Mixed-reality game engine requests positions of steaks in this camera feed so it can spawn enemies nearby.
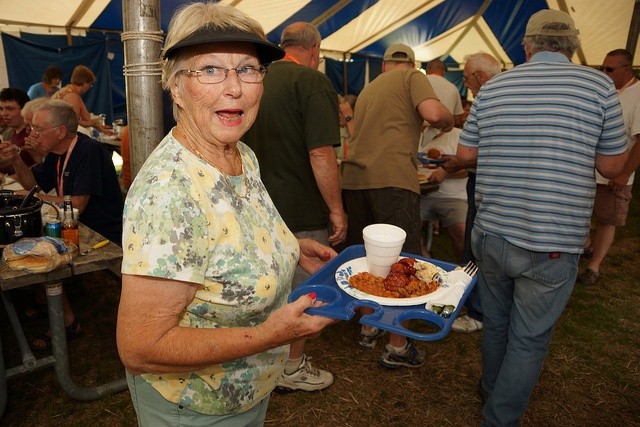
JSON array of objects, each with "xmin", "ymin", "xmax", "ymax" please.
[{"xmin": 349, "ymin": 272, "xmax": 407, "ymax": 298}]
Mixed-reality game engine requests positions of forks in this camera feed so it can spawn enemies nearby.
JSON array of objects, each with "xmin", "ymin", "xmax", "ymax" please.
[{"xmin": 442, "ymin": 261, "xmax": 478, "ymax": 317}]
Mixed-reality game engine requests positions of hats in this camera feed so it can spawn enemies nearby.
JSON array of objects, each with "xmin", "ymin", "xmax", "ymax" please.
[
  {"xmin": 525, "ymin": 10, "xmax": 580, "ymax": 37},
  {"xmin": 163, "ymin": 21, "xmax": 286, "ymax": 64},
  {"xmin": 383, "ymin": 42, "xmax": 414, "ymax": 63}
]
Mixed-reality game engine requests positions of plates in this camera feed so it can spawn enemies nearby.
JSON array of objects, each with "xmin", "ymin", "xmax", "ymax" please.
[{"xmin": 335, "ymin": 256, "xmax": 450, "ymax": 307}]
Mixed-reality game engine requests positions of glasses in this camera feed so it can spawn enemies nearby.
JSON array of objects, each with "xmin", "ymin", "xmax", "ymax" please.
[
  {"xmin": 31, "ymin": 125, "xmax": 60, "ymax": 135},
  {"xmin": 88, "ymin": 82, "xmax": 94, "ymax": 88},
  {"xmin": 175, "ymin": 64, "xmax": 269, "ymax": 83},
  {"xmin": 462, "ymin": 71, "xmax": 477, "ymax": 82},
  {"xmin": 600, "ymin": 64, "xmax": 630, "ymax": 72},
  {"xmin": 318, "ymin": 47, "xmax": 325, "ymax": 64}
]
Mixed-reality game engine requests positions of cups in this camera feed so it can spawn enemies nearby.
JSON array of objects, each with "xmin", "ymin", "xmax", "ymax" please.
[{"xmin": 362, "ymin": 223, "xmax": 406, "ymax": 279}]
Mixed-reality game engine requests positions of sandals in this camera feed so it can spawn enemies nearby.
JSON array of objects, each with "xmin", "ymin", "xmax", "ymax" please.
[
  {"xmin": 25, "ymin": 298, "xmax": 47, "ymax": 319},
  {"xmin": 583, "ymin": 269, "xmax": 598, "ymax": 285},
  {"xmin": 31, "ymin": 317, "xmax": 81, "ymax": 350}
]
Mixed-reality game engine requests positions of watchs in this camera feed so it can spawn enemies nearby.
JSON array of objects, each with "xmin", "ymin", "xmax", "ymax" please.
[{"xmin": 344, "ymin": 115, "xmax": 353, "ymax": 123}]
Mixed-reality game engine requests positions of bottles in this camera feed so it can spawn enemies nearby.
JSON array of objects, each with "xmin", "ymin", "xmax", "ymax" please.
[{"xmin": 61, "ymin": 195, "xmax": 79, "ymax": 251}]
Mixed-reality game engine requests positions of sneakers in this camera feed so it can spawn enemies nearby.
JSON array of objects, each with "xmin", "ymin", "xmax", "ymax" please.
[
  {"xmin": 273, "ymin": 354, "xmax": 333, "ymax": 393},
  {"xmin": 380, "ymin": 341, "xmax": 427, "ymax": 369},
  {"xmin": 359, "ymin": 326, "xmax": 386, "ymax": 347},
  {"xmin": 451, "ymin": 316, "xmax": 483, "ymax": 333}
]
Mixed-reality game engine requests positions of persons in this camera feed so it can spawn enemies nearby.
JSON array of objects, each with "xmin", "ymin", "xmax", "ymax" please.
[
  {"xmin": 444, "ymin": 54, "xmax": 501, "ymax": 333},
  {"xmin": 1, "ymin": 99, "xmax": 124, "ymax": 350},
  {"xmin": 50, "ymin": 65, "xmax": 112, "ymax": 137},
  {"xmin": 423, "ymin": 59, "xmax": 464, "ymax": 127},
  {"xmin": 339, "ymin": 45, "xmax": 455, "ymax": 366},
  {"xmin": 336, "ymin": 93, "xmax": 357, "ymax": 138},
  {"xmin": 461, "ymin": 9, "xmax": 629, "ymax": 426},
  {"xmin": 461, "ymin": 100, "xmax": 473, "ymax": 121},
  {"xmin": 577, "ymin": 50, "xmax": 638, "ymax": 293},
  {"xmin": 27, "ymin": 65, "xmax": 64, "ymax": 100},
  {"xmin": 1, "ymin": 87, "xmax": 33, "ymax": 165},
  {"xmin": 238, "ymin": 21, "xmax": 348, "ymax": 391},
  {"xmin": 115, "ymin": 3, "xmax": 344, "ymax": 427},
  {"xmin": 23, "ymin": 98, "xmax": 50, "ymax": 161},
  {"xmin": 417, "ymin": 126, "xmax": 469, "ymax": 265}
]
